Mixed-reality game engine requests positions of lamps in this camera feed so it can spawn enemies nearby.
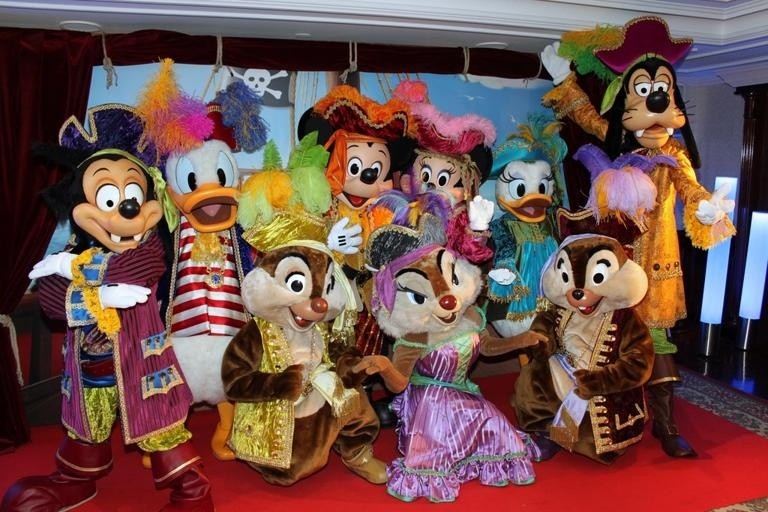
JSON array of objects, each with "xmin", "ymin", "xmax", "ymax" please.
[{"xmin": 699, "ymin": 173, "xmax": 768, "ymax": 358}]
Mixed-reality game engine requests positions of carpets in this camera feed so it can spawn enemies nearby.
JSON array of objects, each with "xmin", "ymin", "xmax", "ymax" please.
[{"xmin": 0, "ymin": 359, "xmax": 767, "ymax": 510}]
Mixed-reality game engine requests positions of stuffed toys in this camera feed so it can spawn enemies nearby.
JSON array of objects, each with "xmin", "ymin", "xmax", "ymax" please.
[{"xmin": 0, "ymin": 14, "xmax": 737, "ymax": 512}]
